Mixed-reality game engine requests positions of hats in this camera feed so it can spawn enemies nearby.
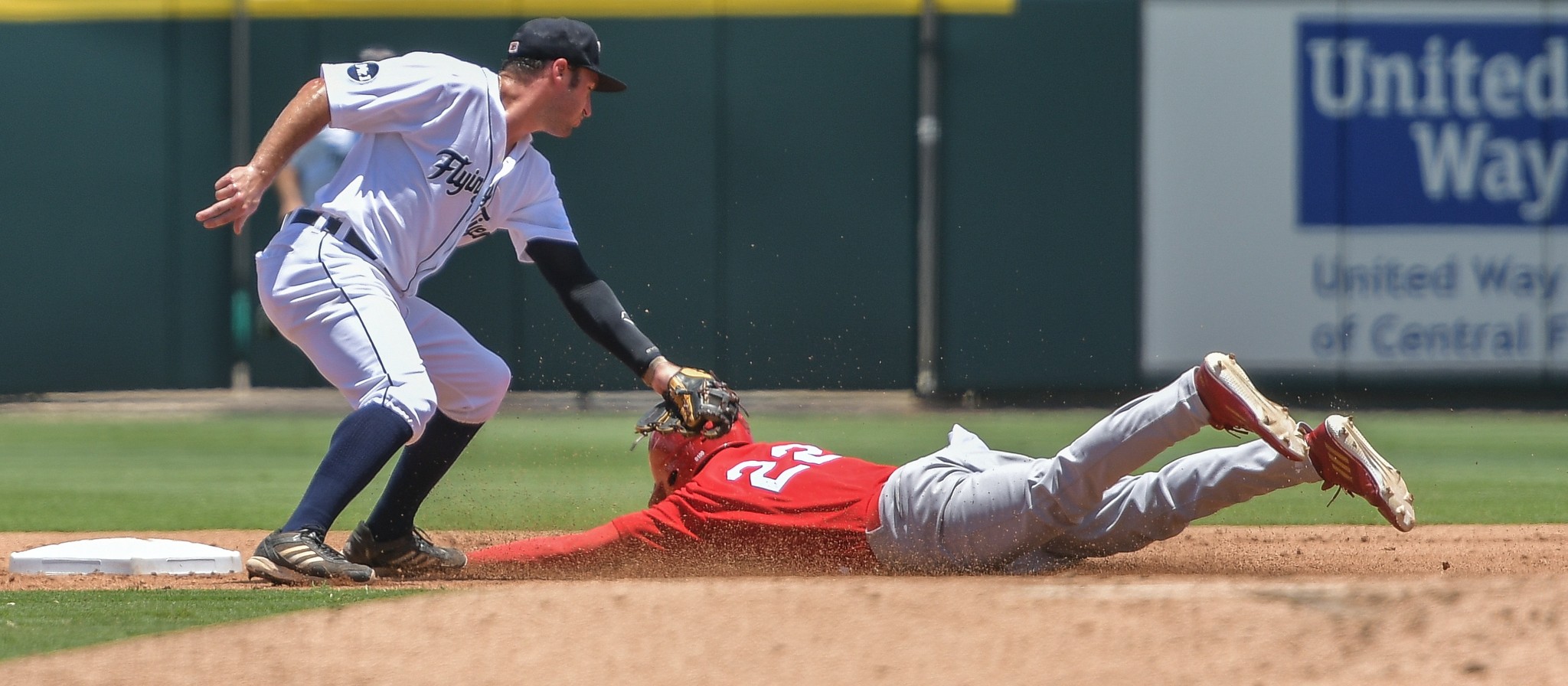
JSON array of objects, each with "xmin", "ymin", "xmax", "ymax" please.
[{"xmin": 509, "ymin": 14, "xmax": 626, "ymax": 93}]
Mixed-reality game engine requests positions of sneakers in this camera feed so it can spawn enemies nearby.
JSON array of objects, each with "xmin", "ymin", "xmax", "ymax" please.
[
  {"xmin": 1303, "ymin": 413, "xmax": 1415, "ymax": 530},
  {"xmin": 342, "ymin": 517, "xmax": 468, "ymax": 571},
  {"xmin": 1195, "ymin": 352, "xmax": 1310, "ymax": 462},
  {"xmin": 245, "ymin": 531, "xmax": 377, "ymax": 585}
]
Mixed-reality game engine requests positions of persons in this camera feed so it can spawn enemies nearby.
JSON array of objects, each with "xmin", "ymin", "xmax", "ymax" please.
[
  {"xmin": 194, "ymin": 15, "xmax": 737, "ymax": 585},
  {"xmin": 441, "ymin": 352, "xmax": 1416, "ymax": 582}
]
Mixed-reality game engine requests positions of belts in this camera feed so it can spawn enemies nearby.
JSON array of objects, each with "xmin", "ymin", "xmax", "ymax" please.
[
  {"xmin": 292, "ymin": 209, "xmax": 376, "ymax": 262},
  {"xmin": 864, "ymin": 484, "xmax": 883, "ymax": 532}
]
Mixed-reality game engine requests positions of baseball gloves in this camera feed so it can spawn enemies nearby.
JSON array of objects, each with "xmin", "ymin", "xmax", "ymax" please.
[{"xmin": 635, "ymin": 367, "xmax": 740, "ymax": 440}]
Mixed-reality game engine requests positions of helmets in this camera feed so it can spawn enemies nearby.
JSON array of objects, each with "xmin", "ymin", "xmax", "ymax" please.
[{"xmin": 648, "ymin": 403, "xmax": 751, "ymax": 507}]
